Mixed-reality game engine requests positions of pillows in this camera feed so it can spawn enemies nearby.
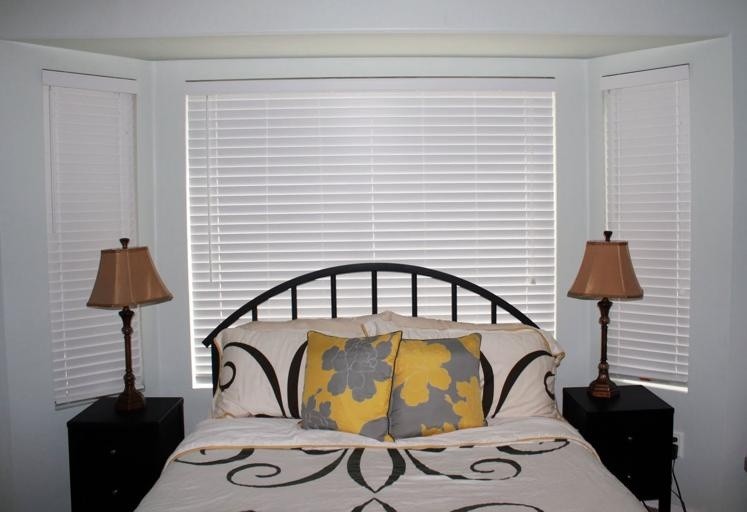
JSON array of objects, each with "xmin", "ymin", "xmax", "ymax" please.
[
  {"xmin": 387, "ymin": 331, "xmax": 488, "ymax": 440},
  {"xmin": 209, "ymin": 310, "xmax": 391, "ymax": 419},
  {"xmin": 390, "ymin": 312, "xmax": 566, "ymax": 420},
  {"xmin": 299, "ymin": 330, "xmax": 404, "ymax": 443}
]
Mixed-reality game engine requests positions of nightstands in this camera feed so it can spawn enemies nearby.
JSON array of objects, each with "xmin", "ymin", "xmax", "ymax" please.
[
  {"xmin": 563, "ymin": 384, "xmax": 675, "ymax": 512},
  {"xmin": 66, "ymin": 397, "xmax": 186, "ymax": 512}
]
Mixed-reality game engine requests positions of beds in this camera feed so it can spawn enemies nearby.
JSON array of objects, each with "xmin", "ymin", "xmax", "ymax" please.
[{"xmin": 134, "ymin": 262, "xmax": 653, "ymax": 512}]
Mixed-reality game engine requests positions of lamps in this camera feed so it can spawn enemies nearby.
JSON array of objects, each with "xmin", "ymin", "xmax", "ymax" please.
[
  {"xmin": 85, "ymin": 238, "xmax": 174, "ymax": 414},
  {"xmin": 567, "ymin": 231, "xmax": 645, "ymax": 399}
]
